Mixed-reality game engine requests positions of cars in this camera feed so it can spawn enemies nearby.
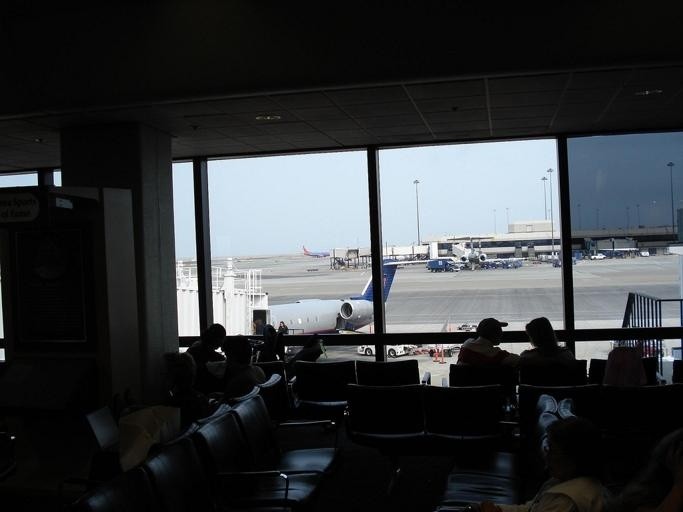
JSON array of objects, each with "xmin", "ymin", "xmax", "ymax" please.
[
  {"xmin": 357, "ymin": 340, "xmax": 416, "ymax": 358},
  {"xmin": 428, "ymin": 344, "xmax": 456, "ymax": 357}
]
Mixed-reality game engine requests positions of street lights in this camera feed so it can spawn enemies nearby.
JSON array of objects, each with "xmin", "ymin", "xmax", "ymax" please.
[
  {"xmin": 627, "ymin": 207, "xmax": 630, "ymax": 228},
  {"xmin": 542, "ymin": 176, "xmax": 549, "ymax": 221},
  {"xmin": 597, "ymin": 208, "xmax": 600, "ymax": 230},
  {"xmin": 667, "ymin": 160, "xmax": 676, "ymax": 234},
  {"xmin": 637, "ymin": 204, "xmax": 641, "ymax": 226},
  {"xmin": 578, "ymin": 203, "xmax": 581, "ymax": 231},
  {"xmin": 547, "ymin": 167, "xmax": 554, "ymax": 258},
  {"xmin": 413, "ymin": 179, "xmax": 422, "ymax": 248}
]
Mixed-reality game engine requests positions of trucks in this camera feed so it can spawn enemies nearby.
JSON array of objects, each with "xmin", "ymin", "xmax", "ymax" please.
[{"xmin": 429, "ymin": 259, "xmax": 460, "ymax": 272}]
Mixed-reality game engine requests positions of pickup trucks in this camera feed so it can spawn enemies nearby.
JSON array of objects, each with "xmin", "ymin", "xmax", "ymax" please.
[{"xmin": 592, "ymin": 254, "xmax": 606, "ymax": 260}]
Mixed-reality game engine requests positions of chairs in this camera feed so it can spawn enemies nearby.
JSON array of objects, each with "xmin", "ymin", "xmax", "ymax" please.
[
  {"xmin": 232, "ymin": 385, "xmax": 260, "ymax": 403},
  {"xmin": 196, "ymin": 403, "xmax": 235, "ymax": 422},
  {"xmin": 448, "ymin": 361, "xmax": 516, "ymax": 384},
  {"xmin": 672, "ymin": 355, "xmax": 683, "ymax": 385},
  {"xmin": 198, "ymin": 411, "xmax": 320, "ymax": 505},
  {"xmin": 161, "ymin": 421, "xmax": 200, "ymax": 448},
  {"xmin": 257, "ymin": 371, "xmax": 284, "ymax": 390},
  {"xmin": 346, "ymin": 381, "xmax": 433, "ymax": 434},
  {"xmin": 516, "ymin": 357, "xmax": 586, "ymax": 383},
  {"xmin": 588, "ymin": 355, "xmax": 661, "ymax": 383},
  {"xmin": 235, "ymin": 394, "xmax": 339, "ymax": 473},
  {"xmin": 294, "ymin": 359, "xmax": 356, "ymax": 409},
  {"xmin": 431, "ymin": 382, "xmax": 508, "ymax": 433},
  {"xmin": 518, "ymin": 381, "xmax": 600, "ymax": 438},
  {"xmin": 85, "ymin": 458, "xmax": 175, "ymax": 512},
  {"xmin": 146, "ymin": 430, "xmax": 293, "ymax": 512},
  {"xmin": 601, "ymin": 382, "xmax": 683, "ymax": 439},
  {"xmin": 356, "ymin": 359, "xmax": 432, "ymax": 388},
  {"xmin": 344, "ymin": 429, "xmax": 429, "ymax": 493}
]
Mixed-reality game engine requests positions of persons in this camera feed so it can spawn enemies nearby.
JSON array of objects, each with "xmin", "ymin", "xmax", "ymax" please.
[
  {"xmin": 181, "ymin": 323, "xmax": 226, "ymax": 367},
  {"xmin": 465, "ymin": 417, "xmax": 612, "ymax": 512},
  {"xmin": 253, "ymin": 319, "xmax": 265, "ymax": 333},
  {"xmin": 457, "ymin": 318, "xmax": 519, "ymax": 379},
  {"xmin": 521, "ymin": 317, "xmax": 578, "ymax": 387},
  {"xmin": 278, "ymin": 321, "xmax": 289, "ymax": 335},
  {"xmin": 263, "ymin": 324, "xmax": 285, "ymax": 361},
  {"xmin": 222, "ymin": 335, "xmax": 266, "ymax": 397}
]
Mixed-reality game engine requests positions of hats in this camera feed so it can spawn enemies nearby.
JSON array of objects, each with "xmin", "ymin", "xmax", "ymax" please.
[{"xmin": 476, "ymin": 317, "xmax": 508, "ymax": 333}]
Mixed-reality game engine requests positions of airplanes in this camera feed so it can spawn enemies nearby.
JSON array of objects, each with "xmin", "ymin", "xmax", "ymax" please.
[
  {"xmin": 384, "ymin": 248, "xmax": 528, "ymax": 273},
  {"xmin": 303, "ymin": 248, "xmax": 329, "ymax": 260},
  {"xmin": 252, "ymin": 258, "xmax": 398, "ymax": 354}
]
